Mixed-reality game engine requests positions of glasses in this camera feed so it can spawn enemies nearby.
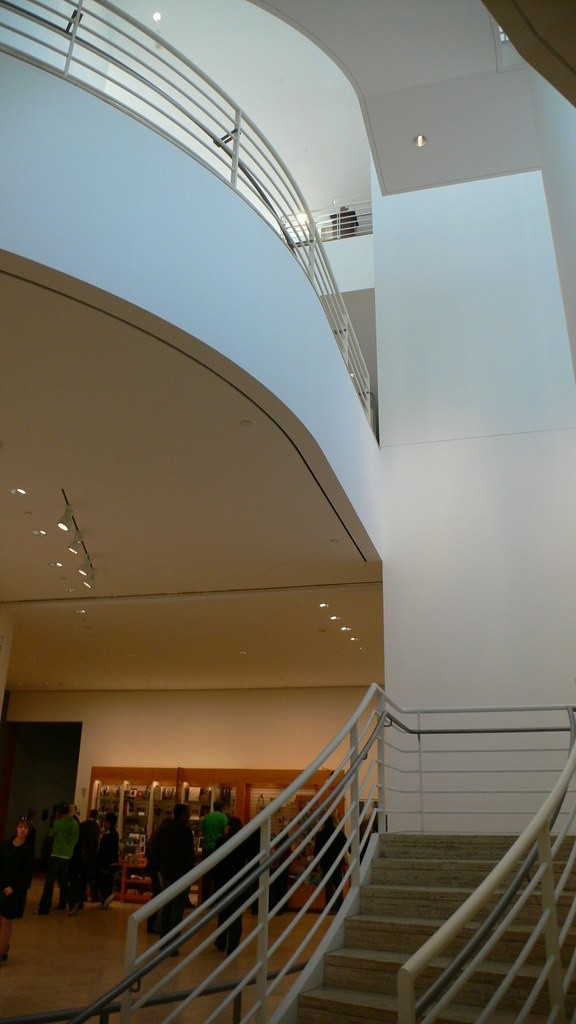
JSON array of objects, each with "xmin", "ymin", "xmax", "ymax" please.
[{"xmin": 18, "ymin": 816, "xmax": 33, "ymax": 825}]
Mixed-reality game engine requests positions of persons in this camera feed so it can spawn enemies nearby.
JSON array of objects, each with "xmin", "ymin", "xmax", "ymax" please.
[
  {"xmin": 38, "ymin": 801, "xmax": 81, "ymax": 913},
  {"xmin": 349, "ymin": 820, "xmax": 380, "ymax": 864},
  {"xmin": 157, "ymin": 804, "xmax": 194, "ymax": 956},
  {"xmin": 214, "ymin": 817, "xmax": 252, "ymax": 957},
  {"xmin": 199, "ymin": 800, "xmax": 229, "ymax": 910},
  {"xmin": 315, "ymin": 816, "xmax": 352, "ymax": 913},
  {"xmin": 79, "ymin": 809, "xmax": 102, "ymax": 903},
  {"xmin": 0, "ymin": 816, "xmax": 36, "ymax": 961},
  {"xmin": 89, "ymin": 813, "xmax": 119, "ymax": 907}
]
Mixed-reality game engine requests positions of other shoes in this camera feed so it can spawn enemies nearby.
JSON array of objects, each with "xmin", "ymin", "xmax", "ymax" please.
[
  {"xmin": 78, "ymin": 907, "xmax": 83, "ymax": 912},
  {"xmin": 214, "ymin": 939, "xmax": 230, "ymax": 958},
  {"xmin": 185, "ymin": 902, "xmax": 196, "ymax": 907},
  {"xmin": 327, "ymin": 907, "xmax": 339, "ymax": 915},
  {"xmin": 164, "ymin": 949, "xmax": 179, "ymax": 956},
  {"xmin": 69, "ymin": 901, "xmax": 79, "ymax": 915},
  {"xmin": 51, "ymin": 906, "xmax": 65, "ymax": 911},
  {"xmin": 251, "ymin": 906, "xmax": 282, "ymax": 916},
  {"xmin": 100, "ymin": 893, "xmax": 115, "ymax": 908},
  {"xmin": 34, "ymin": 910, "xmax": 39, "ymax": 914},
  {"xmin": 146, "ymin": 926, "xmax": 161, "ymax": 933}
]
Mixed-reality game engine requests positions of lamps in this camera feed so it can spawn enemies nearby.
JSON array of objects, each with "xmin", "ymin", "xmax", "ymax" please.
[{"xmin": 56, "ymin": 487, "xmax": 96, "ymax": 589}]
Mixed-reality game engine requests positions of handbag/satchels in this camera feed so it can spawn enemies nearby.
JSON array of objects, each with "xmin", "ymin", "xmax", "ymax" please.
[{"xmin": 343, "ymin": 848, "xmax": 351, "ymax": 865}]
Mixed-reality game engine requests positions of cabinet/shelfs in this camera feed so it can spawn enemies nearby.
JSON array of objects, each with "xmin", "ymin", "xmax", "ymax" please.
[{"xmin": 84, "ymin": 778, "xmax": 222, "ymax": 911}]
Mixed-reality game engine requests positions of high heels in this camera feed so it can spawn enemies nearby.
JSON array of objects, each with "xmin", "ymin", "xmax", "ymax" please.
[{"xmin": 0, "ymin": 944, "xmax": 9, "ymax": 960}]
